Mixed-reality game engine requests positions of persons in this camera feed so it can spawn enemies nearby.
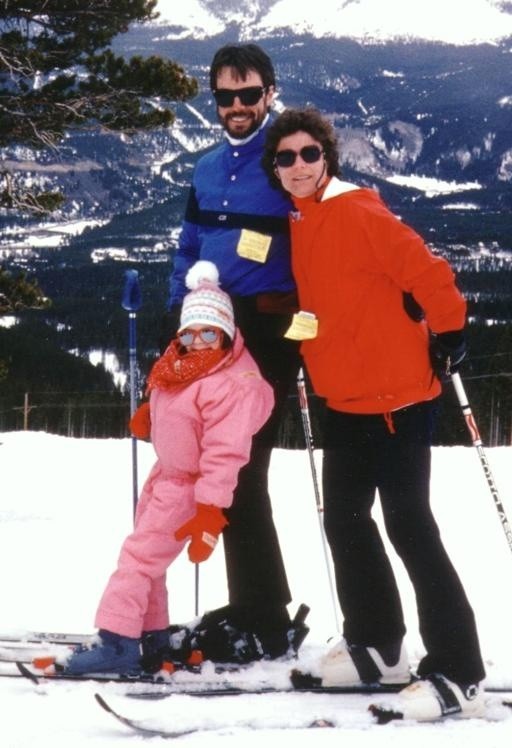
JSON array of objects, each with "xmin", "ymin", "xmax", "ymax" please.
[
  {"xmin": 260, "ymin": 108, "xmax": 484, "ymax": 717},
  {"xmin": 66, "ymin": 261, "xmax": 276, "ymax": 673},
  {"xmin": 169, "ymin": 43, "xmax": 296, "ymax": 652}
]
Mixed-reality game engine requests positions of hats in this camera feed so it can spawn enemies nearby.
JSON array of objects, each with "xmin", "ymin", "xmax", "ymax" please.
[{"xmin": 178, "ymin": 259, "xmax": 237, "ymax": 343}]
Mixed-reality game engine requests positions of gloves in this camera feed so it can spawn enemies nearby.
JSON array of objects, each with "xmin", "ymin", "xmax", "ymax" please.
[
  {"xmin": 174, "ymin": 501, "xmax": 231, "ymax": 567},
  {"xmin": 127, "ymin": 402, "xmax": 153, "ymax": 444},
  {"xmin": 425, "ymin": 327, "xmax": 472, "ymax": 382}
]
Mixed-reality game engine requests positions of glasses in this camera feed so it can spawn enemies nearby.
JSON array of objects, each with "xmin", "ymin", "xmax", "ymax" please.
[
  {"xmin": 270, "ymin": 143, "xmax": 332, "ymax": 167},
  {"xmin": 211, "ymin": 86, "xmax": 268, "ymax": 108},
  {"xmin": 177, "ymin": 328, "xmax": 226, "ymax": 347}
]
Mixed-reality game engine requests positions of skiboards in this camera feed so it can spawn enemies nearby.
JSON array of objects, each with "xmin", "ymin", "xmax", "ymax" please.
[
  {"xmin": 0, "ymin": 634, "xmax": 512, "ymax": 736},
  {"xmin": 94, "ymin": 673, "xmax": 511, "ymax": 737},
  {"xmin": 1, "ymin": 634, "xmax": 504, "ymax": 682}
]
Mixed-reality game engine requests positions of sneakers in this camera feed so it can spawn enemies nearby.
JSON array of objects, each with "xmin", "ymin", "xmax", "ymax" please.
[
  {"xmin": 290, "ymin": 636, "xmax": 414, "ymax": 692},
  {"xmin": 368, "ymin": 671, "xmax": 488, "ymax": 723},
  {"xmin": 138, "ymin": 624, "xmax": 176, "ymax": 669},
  {"xmin": 60, "ymin": 629, "xmax": 143, "ymax": 676}
]
